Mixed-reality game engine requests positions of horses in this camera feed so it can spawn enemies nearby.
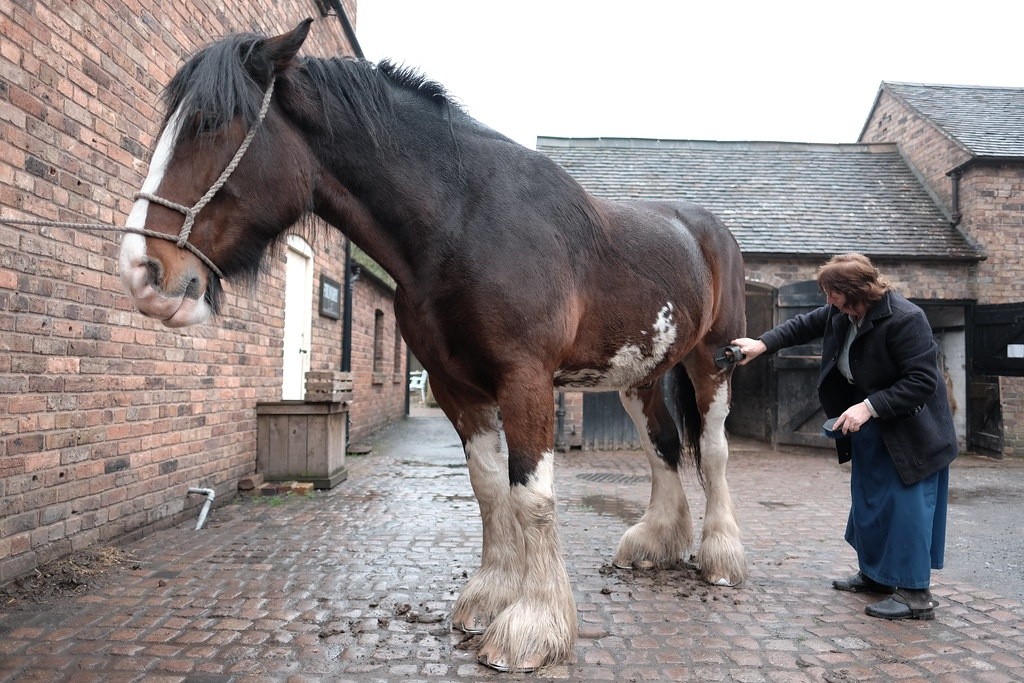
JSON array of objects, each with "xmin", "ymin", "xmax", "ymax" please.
[{"xmin": 115, "ymin": 16, "xmax": 748, "ymax": 672}]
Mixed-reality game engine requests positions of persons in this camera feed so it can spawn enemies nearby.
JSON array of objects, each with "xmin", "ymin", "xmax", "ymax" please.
[{"xmin": 723, "ymin": 254, "xmax": 960, "ymax": 621}]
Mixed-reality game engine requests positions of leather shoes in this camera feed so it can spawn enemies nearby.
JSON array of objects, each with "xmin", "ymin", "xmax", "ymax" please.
[
  {"xmin": 864, "ymin": 587, "xmax": 936, "ymax": 621},
  {"xmin": 832, "ymin": 570, "xmax": 896, "ymax": 596}
]
